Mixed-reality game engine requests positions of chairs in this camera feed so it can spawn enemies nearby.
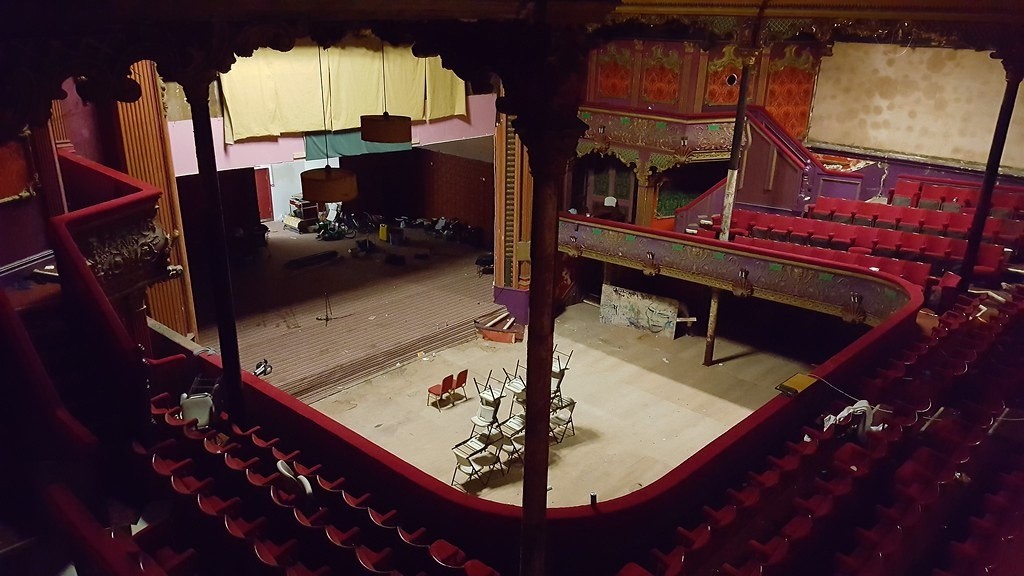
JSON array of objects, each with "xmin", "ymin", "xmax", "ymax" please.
[{"xmin": 107, "ymin": 180, "xmax": 1024, "ymax": 576}]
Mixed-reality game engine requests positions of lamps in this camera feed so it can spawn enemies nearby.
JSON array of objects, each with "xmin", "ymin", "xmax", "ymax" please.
[
  {"xmin": 361, "ymin": 41, "xmax": 412, "ymax": 144},
  {"xmin": 301, "ymin": 46, "xmax": 358, "ymax": 203}
]
